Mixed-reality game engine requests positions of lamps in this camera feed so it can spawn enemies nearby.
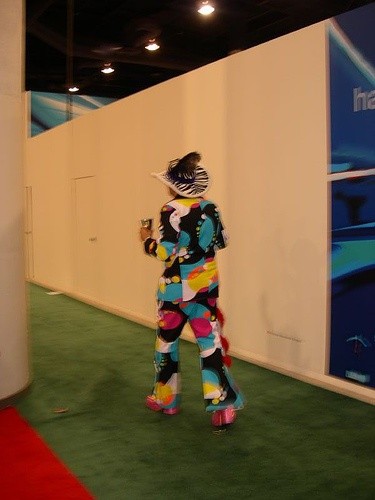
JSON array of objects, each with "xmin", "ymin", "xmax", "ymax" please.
[
  {"xmin": 197, "ymin": 0, "xmax": 216, "ymax": 17},
  {"xmin": 145, "ymin": 38, "xmax": 161, "ymax": 51},
  {"xmin": 68, "ymin": 81, "xmax": 80, "ymax": 93},
  {"xmin": 100, "ymin": 60, "xmax": 115, "ymax": 75}
]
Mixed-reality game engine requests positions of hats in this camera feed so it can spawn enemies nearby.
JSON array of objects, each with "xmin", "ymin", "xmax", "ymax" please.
[{"xmin": 150, "ymin": 150, "xmax": 211, "ymax": 198}]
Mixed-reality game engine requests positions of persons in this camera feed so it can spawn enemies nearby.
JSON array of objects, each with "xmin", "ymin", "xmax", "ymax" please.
[{"xmin": 141, "ymin": 146, "xmax": 246, "ymax": 430}]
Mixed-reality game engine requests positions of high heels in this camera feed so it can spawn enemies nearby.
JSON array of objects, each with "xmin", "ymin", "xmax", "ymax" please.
[
  {"xmin": 212, "ymin": 405, "xmax": 237, "ymax": 427},
  {"xmin": 145, "ymin": 394, "xmax": 180, "ymax": 414}
]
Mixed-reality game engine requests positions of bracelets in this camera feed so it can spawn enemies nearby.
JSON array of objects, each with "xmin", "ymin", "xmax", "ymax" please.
[{"xmin": 142, "ymin": 236, "xmax": 151, "ymax": 244}]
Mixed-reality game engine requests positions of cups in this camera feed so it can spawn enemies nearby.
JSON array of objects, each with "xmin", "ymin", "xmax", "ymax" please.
[{"xmin": 141, "ymin": 218, "xmax": 153, "ymax": 232}]
